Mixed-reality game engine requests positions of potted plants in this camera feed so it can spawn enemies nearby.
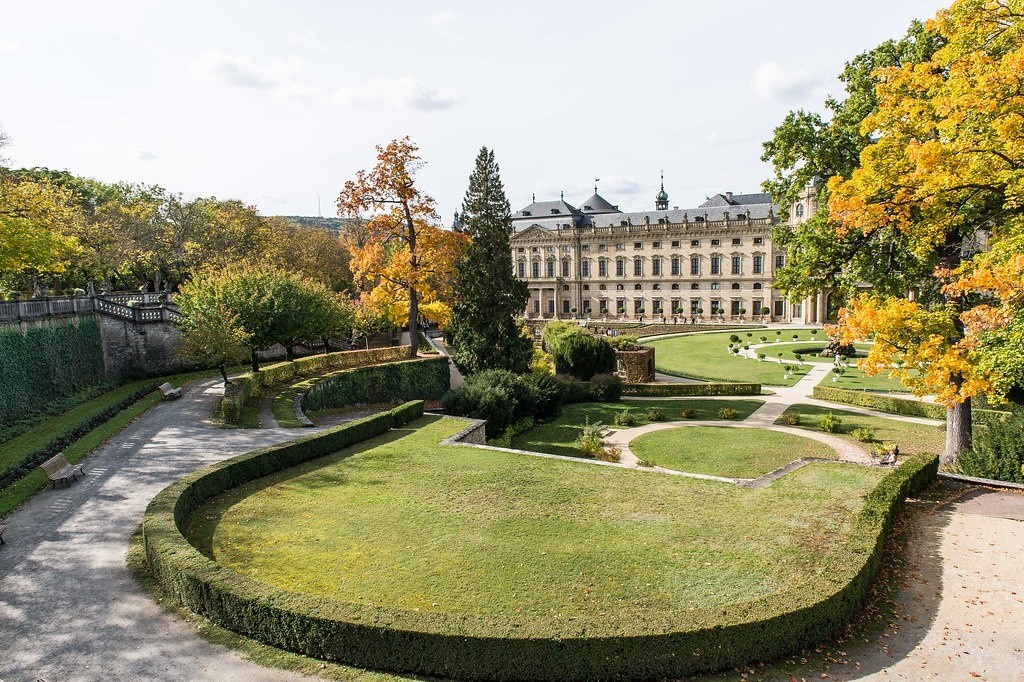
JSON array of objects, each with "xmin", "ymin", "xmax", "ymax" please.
[
  {"xmin": 658, "ymin": 309, "xmax": 664, "ymax": 322},
  {"xmin": 792, "ymin": 335, "xmax": 798, "ymax": 341},
  {"xmin": 601, "ymin": 308, "xmax": 607, "ymax": 322},
  {"xmin": 784, "ymin": 353, "xmax": 805, "ymax": 380},
  {"xmin": 757, "ymin": 353, "xmax": 766, "ymax": 362},
  {"xmin": 718, "ymin": 309, "xmax": 725, "ymax": 322},
  {"xmin": 776, "ymin": 353, "xmax": 783, "ymax": 365},
  {"xmin": 619, "ymin": 307, "xmax": 625, "ymax": 323},
  {"xmin": 729, "ymin": 332, "xmax": 752, "ymax": 359},
  {"xmin": 760, "ymin": 307, "xmax": 770, "ymax": 324},
  {"xmin": 571, "ymin": 307, "xmax": 578, "ymax": 320},
  {"xmin": 638, "ymin": 308, "xmax": 645, "ymax": 321},
  {"xmin": 585, "ymin": 307, "xmax": 592, "ymax": 321},
  {"xmin": 810, "ymin": 329, "xmax": 817, "ymax": 340},
  {"xmin": 775, "ymin": 331, "xmax": 781, "ymax": 342},
  {"xmin": 832, "ymin": 355, "xmax": 849, "ymax": 383},
  {"xmin": 675, "ymin": 307, "xmax": 683, "ymax": 323},
  {"xmin": 760, "ymin": 336, "xmax": 766, "ymax": 344},
  {"xmin": 696, "ymin": 308, "xmax": 703, "ymax": 323},
  {"xmin": 738, "ymin": 308, "xmax": 746, "ymax": 323}
]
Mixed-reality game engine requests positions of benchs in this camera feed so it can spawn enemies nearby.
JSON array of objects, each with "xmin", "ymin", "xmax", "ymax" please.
[
  {"xmin": 38, "ymin": 451, "xmax": 86, "ymax": 490},
  {"xmin": 157, "ymin": 382, "xmax": 183, "ymax": 404}
]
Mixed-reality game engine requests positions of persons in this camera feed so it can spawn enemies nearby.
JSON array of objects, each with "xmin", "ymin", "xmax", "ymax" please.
[
  {"xmin": 577, "ymin": 321, "xmax": 623, "ymax": 336},
  {"xmin": 422, "ymin": 320, "xmax": 429, "ymax": 337},
  {"xmin": 633, "ymin": 314, "xmax": 695, "ymax": 324},
  {"xmin": 880, "ymin": 445, "xmax": 899, "ymax": 465},
  {"xmin": 833, "ymin": 348, "xmax": 840, "ymax": 367}
]
